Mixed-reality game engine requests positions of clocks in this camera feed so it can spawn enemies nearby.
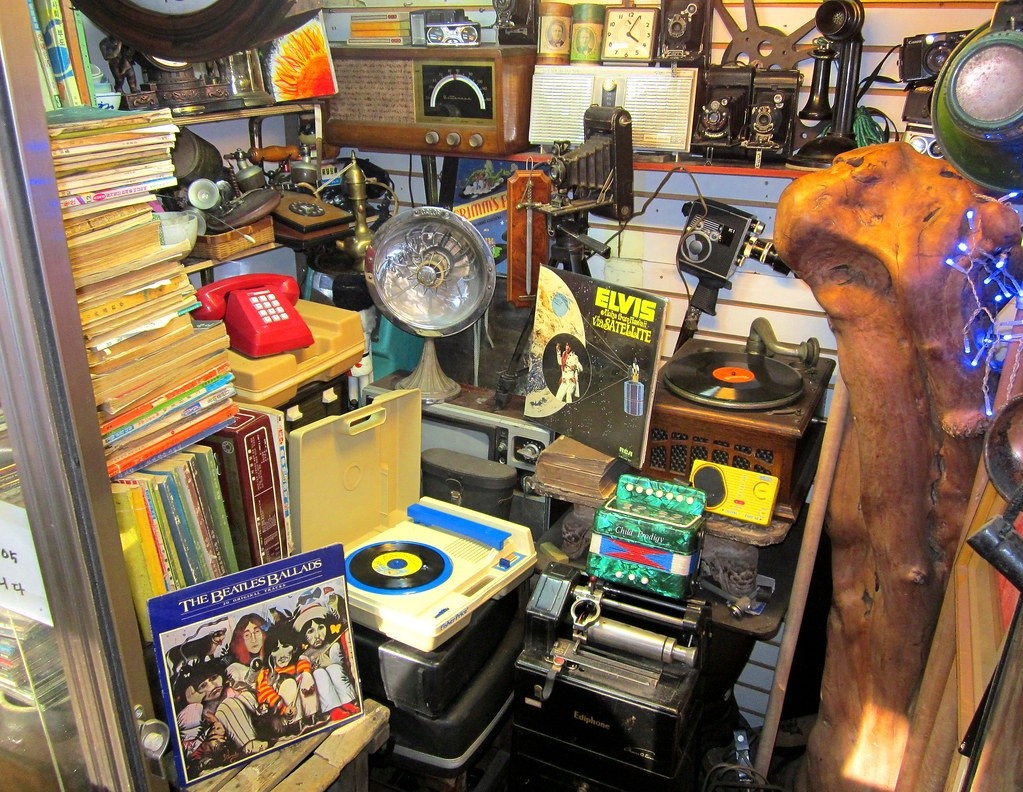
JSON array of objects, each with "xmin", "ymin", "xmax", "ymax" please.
[{"xmin": 600, "ymin": 6, "xmax": 661, "ymax": 63}]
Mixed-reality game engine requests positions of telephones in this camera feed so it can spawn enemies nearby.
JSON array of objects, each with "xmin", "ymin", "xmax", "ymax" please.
[{"xmin": 193, "ymin": 272, "xmax": 315, "ymax": 359}]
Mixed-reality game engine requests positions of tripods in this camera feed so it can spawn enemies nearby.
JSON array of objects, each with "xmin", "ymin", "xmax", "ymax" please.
[{"xmin": 491, "ymin": 199, "xmax": 617, "ymax": 407}]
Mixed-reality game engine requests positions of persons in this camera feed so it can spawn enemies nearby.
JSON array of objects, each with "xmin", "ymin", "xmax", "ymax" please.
[
  {"xmin": 555, "ymin": 338, "xmax": 583, "ymax": 404},
  {"xmin": 548, "ymin": 24, "xmax": 564, "ymax": 48},
  {"xmin": 577, "ymin": 29, "xmax": 592, "ymax": 55}
]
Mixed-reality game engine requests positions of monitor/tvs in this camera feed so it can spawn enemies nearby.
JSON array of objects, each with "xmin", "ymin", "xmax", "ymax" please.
[{"xmin": 358, "ymin": 368, "xmax": 559, "ymax": 545}]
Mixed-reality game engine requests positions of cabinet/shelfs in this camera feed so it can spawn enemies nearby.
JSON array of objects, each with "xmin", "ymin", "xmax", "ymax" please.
[{"xmin": 170, "ymin": 98, "xmax": 324, "ymax": 275}]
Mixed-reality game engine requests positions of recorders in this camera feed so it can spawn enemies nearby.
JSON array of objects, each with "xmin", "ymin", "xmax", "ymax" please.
[
  {"xmin": 317, "ymin": 46, "xmax": 537, "ymax": 158},
  {"xmin": 528, "ymin": 65, "xmax": 705, "ymax": 162},
  {"xmin": 423, "ymin": 23, "xmax": 482, "ymax": 47}
]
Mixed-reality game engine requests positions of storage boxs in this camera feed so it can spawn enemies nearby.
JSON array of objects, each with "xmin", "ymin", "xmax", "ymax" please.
[
  {"xmin": 420, "ymin": 448, "xmax": 518, "ymax": 521},
  {"xmin": 228, "ymin": 298, "xmax": 367, "ymax": 408}
]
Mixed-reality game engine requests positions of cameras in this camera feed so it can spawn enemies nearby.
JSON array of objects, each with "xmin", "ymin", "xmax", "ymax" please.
[
  {"xmin": 753, "ymin": 67, "xmax": 804, "ymax": 168},
  {"xmin": 902, "ymin": 124, "xmax": 944, "ymax": 160},
  {"xmin": 676, "ymin": 197, "xmax": 790, "ymax": 316},
  {"xmin": 661, "ymin": 0, "xmax": 714, "ymax": 68},
  {"xmin": 900, "ymin": 27, "xmax": 977, "ymax": 87},
  {"xmin": 691, "ymin": 62, "xmax": 753, "ymax": 168},
  {"xmin": 492, "ymin": 0, "xmax": 540, "ymax": 45},
  {"xmin": 531, "ymin": 103, "xmax": 634, "ymax": 223},
  {"xmin": 902, "ymin": 86, "xmax": 939, "ymax": 123}
]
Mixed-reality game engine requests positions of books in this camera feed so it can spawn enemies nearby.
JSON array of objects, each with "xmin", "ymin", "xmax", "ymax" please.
[
  {"xmin": 27, "ymin": 0, "xmax": 98, "ymax": 112},
  {"xmin": 533, "ymin": 434, "xmax": 627, "ymax": 498},
  {"xmin": 265, "ymin": 15, "xmax": 339, "ymax": 104},
  {"xmin": 62, "ymin": 194, "xmax": 241, "ymax": 482},
  {"xmin": 46, "ymin": 105, "xmax": 179, "ymax": 209},
  {"xmin": 0, "ymin": 412, "xmax": 25, "ymax": 508},
  {"xmin": 0, "ymin": 607, "xmax": 68, "ymax": 712},
  {"xmin": 522, "ymin": 263, "xmax": 669, "ymax": 471},
  {"xmin": 110, "ymin": 402, "xmax": 293, "ymax": 645},
  {"xmin": 346, "ymin": 12, "xmax": 411, "ymax": 46}
]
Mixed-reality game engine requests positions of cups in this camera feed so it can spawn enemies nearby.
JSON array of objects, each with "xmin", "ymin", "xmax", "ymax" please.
[{"xmin": 94, "ymin": 83, "xmax": 111, "ymax": 92}]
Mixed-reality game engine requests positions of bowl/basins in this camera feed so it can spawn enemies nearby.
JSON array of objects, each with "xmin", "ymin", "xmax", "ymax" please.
[
  {"xmin": 89, "ymin": 63, "xmax": 104, "ymax": 85},
  {"xmin": 95, "ymin": 92, "xmax": 121, "ymax": 110},
  {"xmin": 153, "ymin": 211, "xmax": 198, "ymax": 263}
]
